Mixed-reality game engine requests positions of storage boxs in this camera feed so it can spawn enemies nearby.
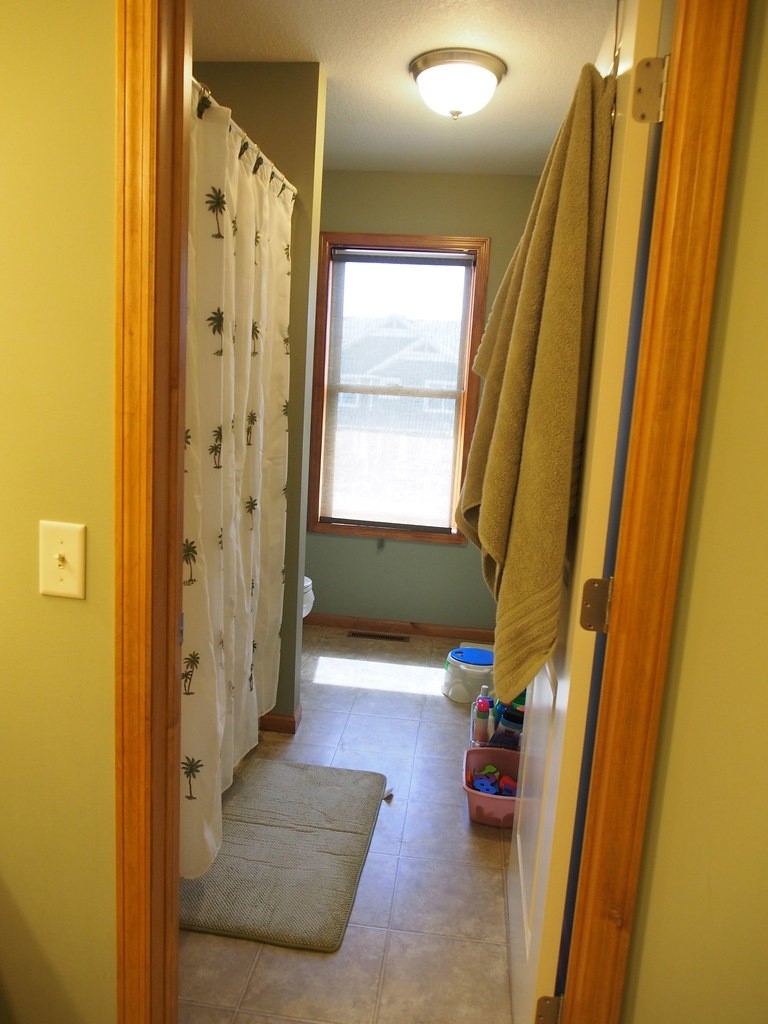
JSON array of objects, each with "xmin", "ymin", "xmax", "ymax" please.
[
  {"xmin": 462, "ymin": 746, "xmax": 525, "ymax": 828},
  {"xmin": 470, "ymin": 702, "xmax": 522, "ymax": 751}
]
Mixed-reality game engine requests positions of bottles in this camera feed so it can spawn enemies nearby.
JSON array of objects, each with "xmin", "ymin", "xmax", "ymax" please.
[{"xmin": 473, "ymin": 684, "xmax": 524, "ymax": 745}]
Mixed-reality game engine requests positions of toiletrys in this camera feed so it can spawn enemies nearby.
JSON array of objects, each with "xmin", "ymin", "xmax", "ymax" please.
[{"xmin": 473, "ymin": 684, "xmax": 526, "ymax": 746}]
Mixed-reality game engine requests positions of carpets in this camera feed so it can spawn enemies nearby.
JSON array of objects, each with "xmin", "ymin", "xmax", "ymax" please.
[{"xmin": 170, "ymin": 756, "xmax": 388, "ymax": 953}]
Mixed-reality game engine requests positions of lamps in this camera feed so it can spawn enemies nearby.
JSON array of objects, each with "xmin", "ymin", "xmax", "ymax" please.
[{"xmin": 409, "ymin": 47, "xmax": 506, "ymax": 122}]
